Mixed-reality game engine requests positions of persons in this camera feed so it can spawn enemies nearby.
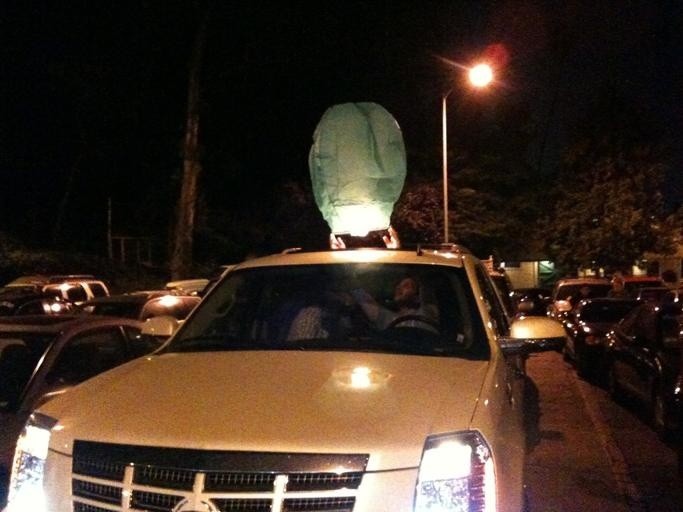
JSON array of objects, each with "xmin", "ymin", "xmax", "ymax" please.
[
  {"xmin": 571, "ymin": 283, "xmax": 595, "ymax": 306},
  {"xmin": 605, "ymin": 274, "xmax": 632, "ymax": 300},
  {"xmin": 330, "ymin": 225, "xmax": 439, "ymax": 334}
]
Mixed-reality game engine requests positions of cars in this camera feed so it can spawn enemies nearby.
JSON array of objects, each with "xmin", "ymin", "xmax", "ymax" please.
[
  {"xmin": 1, "ymin": 312, "xmax": 170, "ymax": 500},
  {"xmin": 1, "ymin": 269, "xmax": 223, "ymax": 349},
  {"xmin": 600, "ymin": 292, "xmax": 682, "ymax": 439},
  {"xmin": 482, "ymin": 255, "xmax": 682, "ymax": 325},
  {"xmin": 557, "ymin": 297, "xmax": 641, "ymax": 380}
]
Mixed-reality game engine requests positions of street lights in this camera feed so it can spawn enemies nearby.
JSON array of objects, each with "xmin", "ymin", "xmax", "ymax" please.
[{"xmin": 440, "ymin": 58, "xmax": 500, "ymax": 251}]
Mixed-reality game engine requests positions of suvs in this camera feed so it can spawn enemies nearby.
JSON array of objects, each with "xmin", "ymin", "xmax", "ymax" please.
[{"xmin": 1, "ymin": 240, "xmax": 569, "ymax": 512}]
